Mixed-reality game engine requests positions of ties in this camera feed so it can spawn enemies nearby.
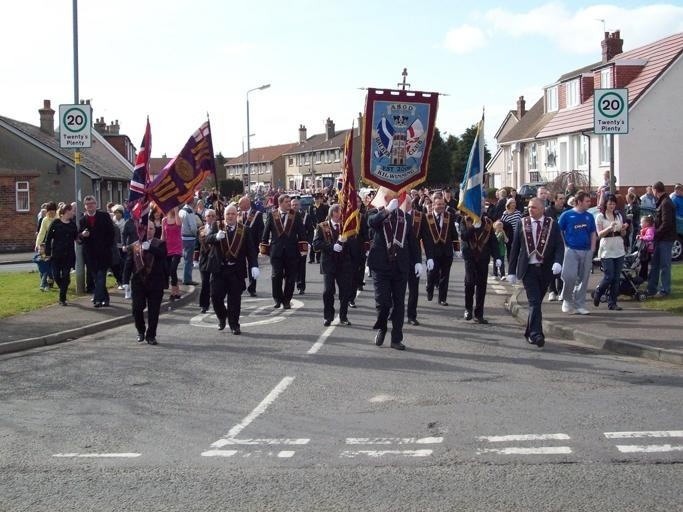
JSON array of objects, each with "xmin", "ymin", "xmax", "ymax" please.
[
  {"xmin": 278, "ymin": 212, "xmax": 287, "ymax": 226},
  {"xmin": 434, "ymin": 212, "xmax": 443, "ymax": 225},
  {"xmin": 333, "ymin": 223, "xmax": 341, "ymax": 234},
  {"xmin": 532, "ymin": 220, "xmax": 542, "ymax": 247}
]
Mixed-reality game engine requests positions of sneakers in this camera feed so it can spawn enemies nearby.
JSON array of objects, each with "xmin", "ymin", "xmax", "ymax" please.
[
  {"xmin": 573, "ymin": 307, "xmax": 592, "ymax": 316},
  {"xmin": 607, "ymin": 303, "xmax": 623, "ymax": 312},
  {"xmin": 547, "ymin": 292, "xmax": 556, "ymax": 301},
  {"xmin": 640, "ymin": 289, "xmax": 658, "ymax": 298},
  {"xmin": 500, "ymin": 276, "xmax": 507, "ymax": 282},
  {"xmin": 561, "ymin": 298, "xmax": 571, "ymax": 313},
  {"xmin": 592, "ymin": 286, "xmax": 602, "ymax": 307},
  {"xmin": 182, "ymin": 278, "xmax": 199, "ymax": 285},
  {"xmin": 652, "ymin": 289, "xmax": 672, "ymax": 299}
]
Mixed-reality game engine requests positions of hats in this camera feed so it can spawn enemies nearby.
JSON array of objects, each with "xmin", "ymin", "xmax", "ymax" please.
[
  {"xmin": 441, "ymin": 186, "xmax": 454, "ymax": 192},
  {"xmin": 311, "ymin": 191, "xmax": 324, "ymax": 199}
]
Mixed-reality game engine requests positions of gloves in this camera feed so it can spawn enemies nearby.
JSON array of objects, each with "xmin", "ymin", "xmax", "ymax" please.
[
  {"xmin": 249, "ymin": 266, "xmax": 260, "ymax": 280},
  {"xmin": 507, "ymin": 274, "xmax": 519, "ymax": 285},
  {"xmin": 412, "ymin": 262, "xmax": 424, "ymax": 279},
  {"xmin": 299, "ymin": 251, "xmax": 308, "ymax": 257},
  {"xmin": 215, "ymin": 229, "xmax": 226, "ymax": 242},
  {"xmin": 140, "ymin": 238, "xmax": 151, "ymax": 251},
  {"xmin": 494, "ymin": 259, "xmax": 502, "ymax": 268},
  {"xmin": 550, "ymin": 261, "xmax": 563, "ymax": 276},
  {"xmin": 425, "ymin": 257, "xmax": 435, "ymax": 272},
  {"xmin": 333, "ymin": 243, "xmax": 343, "ymax": 254},
  {"xmin": 384, "ymin": 197, "xmax": 400, "ymax": 214}
]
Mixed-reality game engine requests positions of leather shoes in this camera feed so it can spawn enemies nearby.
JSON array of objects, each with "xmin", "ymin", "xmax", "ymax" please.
[
  {"xmin": 246, "ymin": 287, "xmax": 258, "ymax": 299},
  {"xmin": 296, "ymin": 287, "xmax": 305, "ymax": 295},
  {"xmin": 34, "ymin": 267, "xmax": 133, "ymax": 308},
  {"xmin": 348, "ymin": 266, "xmax": 373, "ymax": 309},
  {"xmin": 426, "ymin": 291, "xmax": 448, "ymax": 308},
  {"xmin": 321, "ymin": 316, "xmax": 351, "ymax": 327},
  {"xmin": 524, "ymin": 332, "xmax": 546, "ymax": 348},
  {"xmin": 132, "ymin": 327, "xmax": 157, "ymax": 347},
  {"xmin": 199, "ymin": 306, "xmax": 209, "ymax": 315},
  {"xmin": 407, "ymin": 318, "xmax": 420, "ymax": 327},
  {"xmin": 463, "ymin": 309, "xmax": 490, "ymax": 324},
  {"xmin": 271, "ymin": 298, "xmax": 292, "ymax": 309},
  {"xmin": 215, "ymin": 316, "xmax": 243, "ymax": 336},
  {"xmin": 373, "ymin": 323, "xmax": 406, "ymax": 353}
]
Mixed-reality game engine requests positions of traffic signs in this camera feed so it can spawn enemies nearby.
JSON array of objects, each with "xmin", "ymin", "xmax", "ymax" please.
[
  {"xmin": 59, "ymin": 105, "xmax": 91, "ymax": 149},
  {"xmin": 593, "ymin": 89, "xmax": 627, "ymax": 134}
]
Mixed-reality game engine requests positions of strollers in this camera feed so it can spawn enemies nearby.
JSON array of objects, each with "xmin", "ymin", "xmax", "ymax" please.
[{"xmin": 590, "ymin": 234, "xmax": 647, "ymax": 303}]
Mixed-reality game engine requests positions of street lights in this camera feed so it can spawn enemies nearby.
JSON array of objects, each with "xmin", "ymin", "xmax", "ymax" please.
[
  {"xmin": 241, "ymin": 134, "xmax": 255, "ymax": 183},
  {"xmin": 300, "ymin": 139, "xmax": 314, "ymax": 177},
  {"xmin": 245, "ymin": 84, "xmax": 271, "ymax": 192}
]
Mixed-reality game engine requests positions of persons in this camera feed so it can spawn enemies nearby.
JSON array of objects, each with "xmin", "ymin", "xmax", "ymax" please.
[
  {"xmin": 390, "ymin": 193, "xmax": 434, "ymax": 325},
  {"xmin": 120, "ymin": 218, "xmax": 169, "ymax": 346},
  {"xmin": 203, "ymin": 204, "xmax": 260, "ymax": 334},
  {"xmin": 507, "ymin": 197, "xmax": 563, "ymax": 348},
  {"xmin": 366, "ymin": 190, "xmax": 424, "ymax": 350},
  {"xmin": 312, "ymin": 204, "xmax": 354, "ymax": 326},
  {"xmin": 32, "ymin": 170, "xmax": 682, "ymax": 314},
  {"xmin": 454, "ymin": 212, "xmax": 502, "ymax": 323}
]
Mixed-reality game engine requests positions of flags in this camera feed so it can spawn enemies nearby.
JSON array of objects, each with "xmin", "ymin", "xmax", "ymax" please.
[
  {"xmin": 147, "ymin": 121, "xmax": 213, "ymax": 215},
  {"xmin": 338, "ymin": 128, "xmax": 362, "ymax": 240},
  {"xmin": 456, "ymin": 114, "xmax": 484, "ymax": 223},
  {"xmin": 360, "ymin": 86, "xmax": 439, "ymax": 192},
  {"xmin": 128, "ymin": 121, "xmax": 152, "ymax": 203}
]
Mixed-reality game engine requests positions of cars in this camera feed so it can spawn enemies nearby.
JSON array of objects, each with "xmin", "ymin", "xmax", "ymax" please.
[
  {"xmin": 557, "ymin": 205, "xmax": 683, "ymax": 263},
  {"xmin": 300, "ymin": 193, "xmax": 313, "ymax": 210}
]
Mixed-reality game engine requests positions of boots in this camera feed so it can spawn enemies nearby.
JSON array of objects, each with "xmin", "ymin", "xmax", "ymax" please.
[{"xmin": 168, "ymin": 286, "xmax": 182, "ymax": 303}]
